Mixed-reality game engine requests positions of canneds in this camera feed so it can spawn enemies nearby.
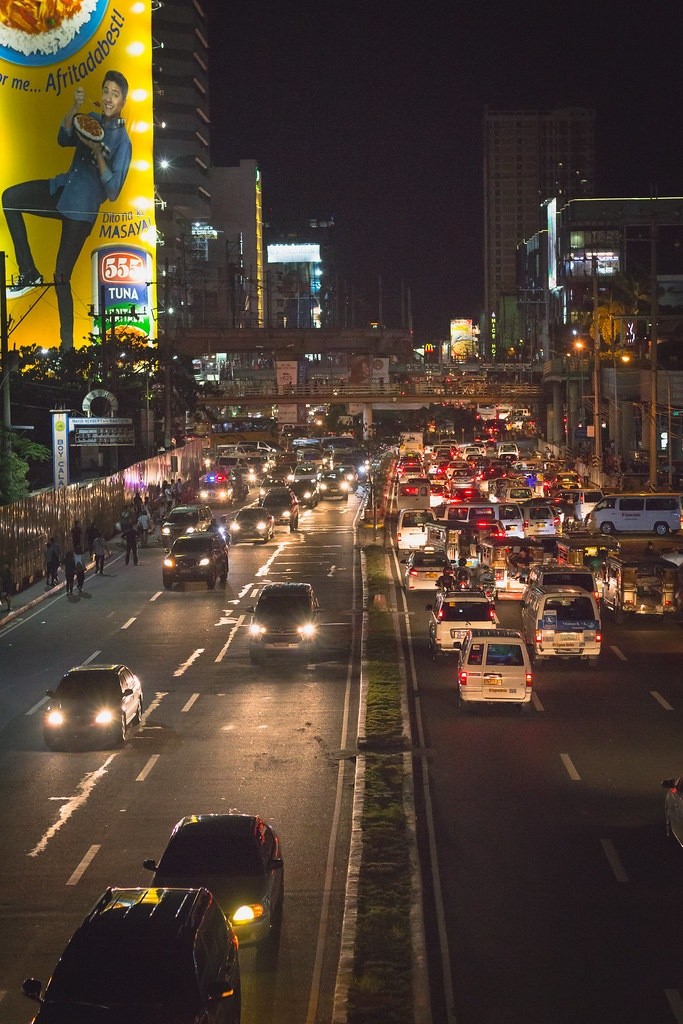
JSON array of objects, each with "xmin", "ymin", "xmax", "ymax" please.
[{"xmin": 91, "ymin": 242, "xmax": 154, "ymax": 373}]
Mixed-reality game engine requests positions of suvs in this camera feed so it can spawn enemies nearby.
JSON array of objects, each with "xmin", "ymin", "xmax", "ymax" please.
[
  {"xmin": 424, "ymin": 589, "xmax": 497, "ymax": 664},
  {"xmin": 20, "ymin": 885, "xmax": 245, "ymax": 1024},
  {"xmin": 161, "ymin": 532, "xmax": 229, "ymax": 589}
]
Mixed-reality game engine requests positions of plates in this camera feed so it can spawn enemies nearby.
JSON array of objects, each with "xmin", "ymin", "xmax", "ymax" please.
[
  {"xmin": 73, "ymin": 113, "xmax": 105, "ymax": 142},
  {"xmin": 0, "ymin": 0, "xmax": 109, "ymax": 66}
]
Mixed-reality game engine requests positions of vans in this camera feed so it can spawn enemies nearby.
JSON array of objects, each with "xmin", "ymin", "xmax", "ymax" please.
[
  {"xmin": 453, "ymin": 629, "xmax": 534, "ymax": 713},
  {"xmin": 520, "ymin": 584, "xmax": 604, "ymax": 666},
  {"xmin": 582, "ymin": 493, "xmax": 683, "ymax": 537}
]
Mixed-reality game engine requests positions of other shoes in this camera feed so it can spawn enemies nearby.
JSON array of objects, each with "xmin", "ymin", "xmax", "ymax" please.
[
  {"xmin": 55, "ymin": 577, "xmax": 58, "ymax": 580},
  {"xmin": 134, "ymin": 563, "xmax": 140, "ymax": 566},
  {"xmin": 95, "ymin": 568, "xmax": 100, "ymax": 574},
  {"xmin": 77, "ymin": 585, "xmax": 83, "ymax": 592}
]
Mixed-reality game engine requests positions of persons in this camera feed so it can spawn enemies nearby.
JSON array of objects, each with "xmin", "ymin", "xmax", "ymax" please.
[
  {"xmin": 583, "ymin": 463, "xmax": 590, "ymax": 485},
  {"xmin": 603, "ymin": 450, "xmax": 611, "ymax": 462},
  {"xmin": 629, "ymin": 457, "xmax": 649, "ymax": 473},
  {"xmin": 85, "ymin": 520, "xmax": 113, "ymax": 576},
  {"xmin": 614, "ymin": 452, "xmax": 626, "ymax": 473},
  {"xmin": 526, "ymin": 475, "xmax": 537, "ymax": 491},
  {"xmin": 64, "ymin": 551, "xmax": 77, "ymax": 595},
  {"xmin": 1, "ymin": 71, "xmax": 130, "ymax": 348},
  {"xmin": 45, "ymin": 537, "xmax": 63, "ymax": 587},
  {"xmin": 70, "ymin": 518, "xmax": 83, "ymax": 554},
  {"xmin": 479, "ymin": 566, "xmax": 495, "ymax": 592},
  {"xmin": 507, "ymin": 547, "xmax": 534, "ymax": 576},
  {"xmin": 163, "ymin": 475, "xmax": 184, "ymax": 514},
  {"xmin": 611, "ymin": 440, "xmax": 618, "ymax": 453},
  {"xmin": 426, "ymin": 376, "xmax": 464, "ymax": 394},
  {"xmin": 454, "ymin": 557, "xmax": 475, "ymax": 578},
  {"xmin": 526, "ymin": 447, "xmax": 557, "ymax": 459},
  {"xmin": 207, "ymin": 518, "xmax": 221, "ymax": 534},
  {"xmin": 0, "ymin": 562, "xmax": 15, "ymax": 610},
  {"xmin": 455, "ymin": 572, "xmax": 473, "ymax": 590},
  {"xmin": 436, "ymin": 566, "xmax": 455, "ymax": 588},
  {"xmin": 119, "ymin": 492, "xmax": 160, "ymax": 567},
  {"xmin": 75, "ymin": 560, "xmax": 88, "ymax": 592}
]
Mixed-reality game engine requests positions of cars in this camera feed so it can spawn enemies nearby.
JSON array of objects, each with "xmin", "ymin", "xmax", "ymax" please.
[
  {"xmin": 661, "ymin": 774, "xmax": 683, "ymax": 849},
  {"xmin": 142, "ymin": 814, "xmax": 285, "ymax": 948},
  {"xmin": 392, "ymin": 403, "xmax": 683, "ymax": 625},
  {"xmin": 228, "ymin": 506, "xmax": 280, "ymax": 545},
  {"xmin": 41, "ymin": 664, "xmax": 144, "ymax": 752},
  {"xmin": 159, "ymin": 504, "xmax": 214, "ymax": 544},
  {"xmin": 245, "ymin": 581, "xmax": 325, "ymax": 664},
  {"xmin": 197, "ymin": 423, "xmax": 387, "ymax": 530}
]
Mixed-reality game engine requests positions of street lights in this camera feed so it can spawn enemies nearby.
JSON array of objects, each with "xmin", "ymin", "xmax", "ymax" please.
[
  {"xmin": 620, "ymin": 354, "xmax": 674, "ymax": 494},
  {"xmin": 87, "ymin": 350, "xmax": 126, "ymax": 395},
  {"xmin": 575, "ymin": 342, "xmax": 620, "ymax": 465},
  {"xmin": 145, "ymin": 354, "xmax": 178, "ymax": 462},
  {"xmin": 0, "ymin": 346, "xmax": 49, "ymax": 455}
]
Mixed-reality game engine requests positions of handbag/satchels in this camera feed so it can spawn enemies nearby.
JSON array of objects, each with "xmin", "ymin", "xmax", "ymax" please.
[{"xmin": 52, "ymin": 549, "xmax": 59, "ymax": 566}]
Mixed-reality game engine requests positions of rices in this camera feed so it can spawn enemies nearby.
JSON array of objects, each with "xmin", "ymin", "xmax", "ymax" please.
[
  {"xmin": 74, "ymin": 116, "xmax": 104, "ymax": 140},
  {"xmin": 0, "ymin": 0, "xmax": 100, "ymax": 56}
]
